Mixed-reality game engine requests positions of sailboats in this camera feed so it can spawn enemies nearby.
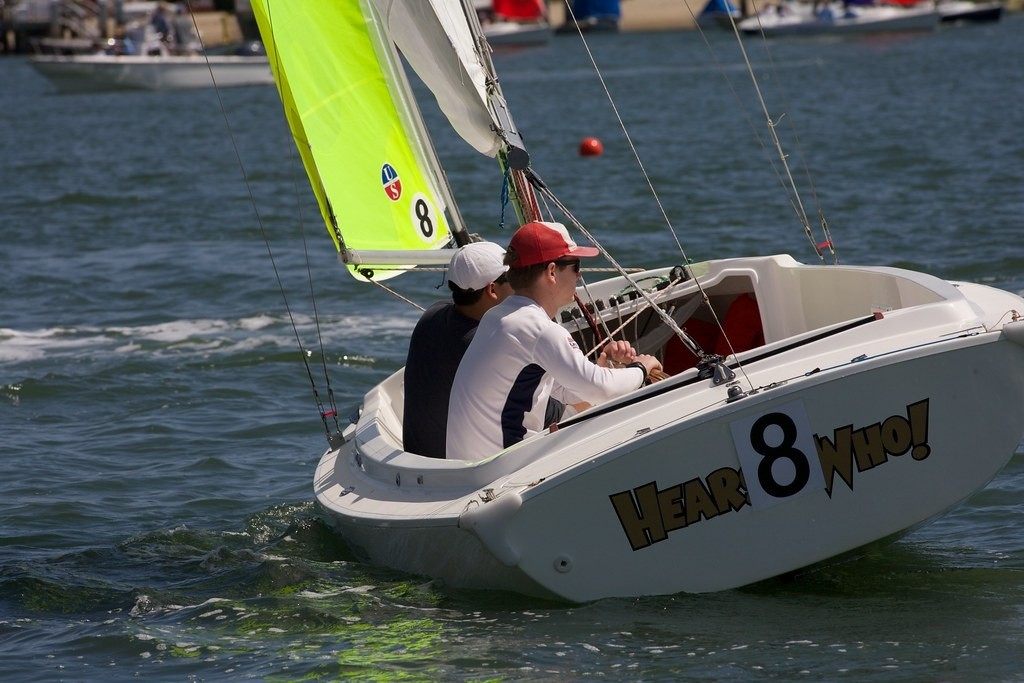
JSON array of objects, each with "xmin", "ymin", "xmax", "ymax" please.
[{"xmin": 168, "ymin": 1, "xmax": 1024, "ymax": 609}]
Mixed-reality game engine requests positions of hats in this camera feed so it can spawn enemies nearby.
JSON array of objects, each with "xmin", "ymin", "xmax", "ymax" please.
[
  {"xmin": 447, "ymin": 241, "xmax": 510, "ymax": 292},
  {"xmin": 507, "ymin": 220, "xmax": 599, "ymax": 267}
]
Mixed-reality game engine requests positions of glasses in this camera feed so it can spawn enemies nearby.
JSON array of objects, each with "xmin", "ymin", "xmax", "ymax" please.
[{"xmin": 544, "ymin": 258, "xmax": 581, "ymax": 272}]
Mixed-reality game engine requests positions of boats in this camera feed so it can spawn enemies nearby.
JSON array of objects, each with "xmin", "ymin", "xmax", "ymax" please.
[{"xmin": 23, "ymin": 0, "xmax": 1024, "ymax": 95}]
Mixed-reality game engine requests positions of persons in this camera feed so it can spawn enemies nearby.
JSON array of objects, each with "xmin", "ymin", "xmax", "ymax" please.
[
  {"xmin": 151, "ymin": 4, "xmax": 191, "ymax": 56},
  {"xmin": 446, "ymin": 220, "xmax": 663, "ymax": 462},
  {"xmin": 403, "ymin": 242, "xmax": 566, "ymax": 459}
]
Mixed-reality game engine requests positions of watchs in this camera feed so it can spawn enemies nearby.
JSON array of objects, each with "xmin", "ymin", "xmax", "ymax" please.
[{"xmin": 626, "ymin": 362, "xmax": 647, "ymax": 388}]
